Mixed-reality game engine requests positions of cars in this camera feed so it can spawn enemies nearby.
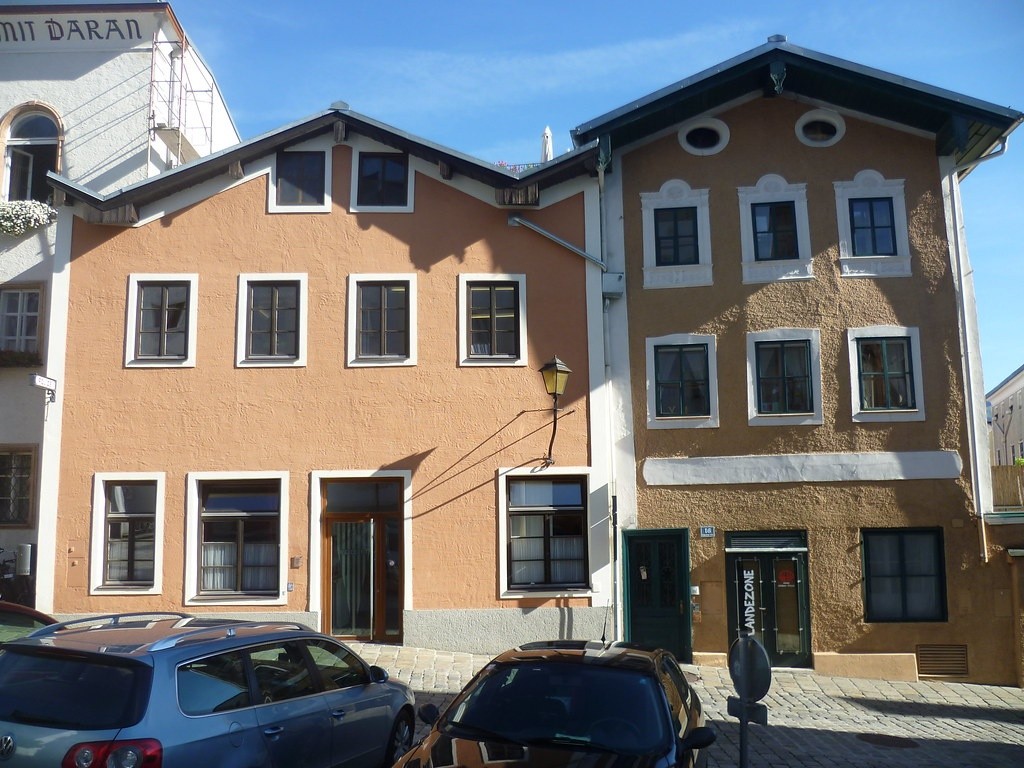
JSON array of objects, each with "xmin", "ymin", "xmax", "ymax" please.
[{"xmin": 394, "ymin": 637, "xmax": 717, "ymax": 768}]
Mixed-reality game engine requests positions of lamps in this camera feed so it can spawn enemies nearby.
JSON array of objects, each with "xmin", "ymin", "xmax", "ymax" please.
[{"xmin": 537, "ymin": 354, "xmax": 574, "ymax": 465}]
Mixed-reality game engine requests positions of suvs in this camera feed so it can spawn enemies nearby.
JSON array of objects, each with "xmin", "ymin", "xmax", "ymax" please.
[{"xmin": 0, "ymin": 610, "xmax": 416, "ymax": 768}]
